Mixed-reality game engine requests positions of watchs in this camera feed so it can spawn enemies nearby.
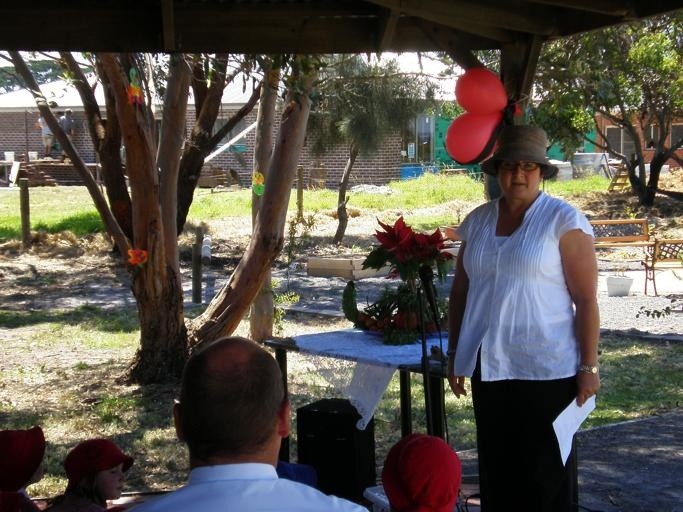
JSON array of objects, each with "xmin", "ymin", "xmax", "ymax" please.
[{"xmin": 576, "ymin": 360, "xmax": 599, "ymax": 376}]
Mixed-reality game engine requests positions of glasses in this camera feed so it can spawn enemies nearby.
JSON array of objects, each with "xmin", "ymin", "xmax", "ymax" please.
[{"xmin": 500, "ymin": 159, "xmax": 538, "ymax": 172}]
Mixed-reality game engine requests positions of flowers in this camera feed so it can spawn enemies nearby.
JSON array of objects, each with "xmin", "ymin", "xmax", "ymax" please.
[{"xmin": 342, "ymin": 216, "xmax": 454, "ymax": 344}]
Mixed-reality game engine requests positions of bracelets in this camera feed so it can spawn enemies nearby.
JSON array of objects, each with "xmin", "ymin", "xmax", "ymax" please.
[{"xmin": 445, "ymin": 348, "xmax": 456, "ymax": 356}]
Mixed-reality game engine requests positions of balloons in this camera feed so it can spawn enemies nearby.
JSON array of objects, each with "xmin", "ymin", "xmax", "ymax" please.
[
  {"xmin": 444, "ymin": 110, "xmax": 505, "ymax": 168},
  {"xmin": 453, "ymin": 65, "xmax": 508, "ymax": 115}
]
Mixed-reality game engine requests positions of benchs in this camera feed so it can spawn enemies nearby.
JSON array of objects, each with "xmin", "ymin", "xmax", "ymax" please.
[{"xmin": 586, "ymin": 219, "xmax": 682, "ymax": 296}]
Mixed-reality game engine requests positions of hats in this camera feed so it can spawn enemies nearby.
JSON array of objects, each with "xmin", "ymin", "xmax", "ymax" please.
[
  {"xmin": 380, "ymin": 431, "xmax": 461, "ymax": 512},
  {"xmin": 64, "ymin": 438, "xmax": 134, "ymax": 489},
  {"xmin": 0, "ymin": 425, "xmax": 45, "ymax": 493},
  {"xmin": 480, "ymin": 125, "xmax": 559, "ymax": 180}
]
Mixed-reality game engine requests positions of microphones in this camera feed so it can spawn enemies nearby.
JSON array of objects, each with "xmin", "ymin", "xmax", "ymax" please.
[{"xmin": 418, "ymin": 265, "xmax": 441, "ymax": 328}]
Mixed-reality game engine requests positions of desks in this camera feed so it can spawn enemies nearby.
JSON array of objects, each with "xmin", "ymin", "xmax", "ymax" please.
[{"xmin": 258, "ymin": 325, "xmax": 449, "ymax": 512}]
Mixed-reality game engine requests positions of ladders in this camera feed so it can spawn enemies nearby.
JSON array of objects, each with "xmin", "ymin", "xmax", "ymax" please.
[{"xmin": 608, "ymin": 159, "xmax": 628, "ymax": 191}]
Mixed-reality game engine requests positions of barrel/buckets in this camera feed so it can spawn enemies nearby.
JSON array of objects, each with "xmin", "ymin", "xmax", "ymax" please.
[
  {"xmin": 28, "ymin": 152, "xmax": 38, "ymax": 160},
  {"xmin": 608, "ymin": 275, "xmax": 633, "ymax": 296},
  {"xmin": 402, "ymin": 163, "xmax": 423, "ymax": 181},
  {"xmin": 4, "ymin": 151, "xmax": 15, "ymax": 161}
]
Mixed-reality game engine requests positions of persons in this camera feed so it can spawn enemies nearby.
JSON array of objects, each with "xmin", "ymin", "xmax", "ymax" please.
[
  {"xmin": 118, "ymin": 334, "xmax": 369, "ymax": 512},
  {"xmin": 445, "ymin": 122, "xmax": 601, "ymax": 511},
  {"xmin": 44, "ymin": 439, "xmax": 134, "ymax": 512},
  {"xmin": 35, "ymin": 115, "xmax": 54, "ymax": 155},
  {"xmin": 56, "ymin": 107, "xmax": 74, "ymax": 163},
  {"xmin": 0, "ymin": 427, "xmax": 48, "ymax": 511}
]
